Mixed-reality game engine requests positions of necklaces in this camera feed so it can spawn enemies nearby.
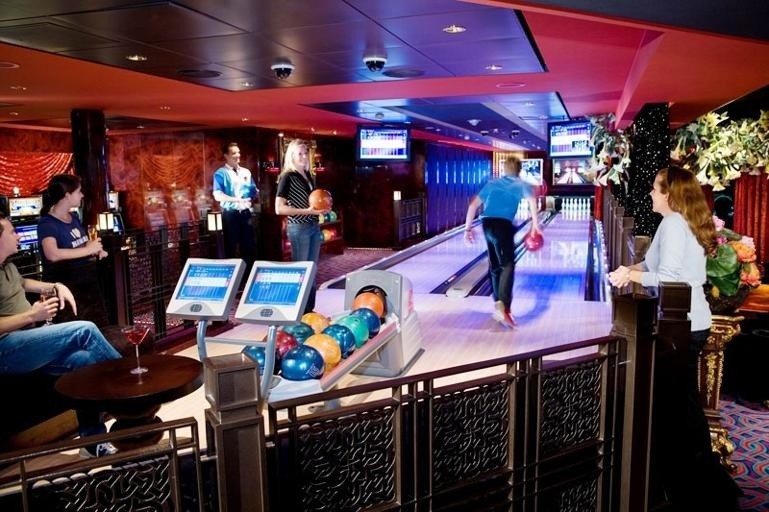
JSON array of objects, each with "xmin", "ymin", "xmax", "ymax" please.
[{"xmin": 60, "ymin": 214, "xmax": 71, "ymax": 222}]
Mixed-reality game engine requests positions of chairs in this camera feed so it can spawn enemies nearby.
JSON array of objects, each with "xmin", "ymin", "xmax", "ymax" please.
[{"xmin": 0, "ymin": 270, "xmax": 152, "ymax": 453}]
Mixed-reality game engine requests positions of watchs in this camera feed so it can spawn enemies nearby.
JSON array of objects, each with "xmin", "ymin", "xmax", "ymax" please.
[
  {"xmin": 52, "ymin": 281, "xmax": 61, "ymax": 292},
  {"xmin": 627, "ymin": 269, "xmax": 633, "ymax": 281}
]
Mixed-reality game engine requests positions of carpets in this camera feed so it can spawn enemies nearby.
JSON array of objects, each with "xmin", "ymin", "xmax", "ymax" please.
[
  {"xmin": 718, "ymin": 396, "xmax": 769, "ymax": 512},
  {"xmin": 131, "ymin": 249, "xmax": 397, "ymax": 325}
]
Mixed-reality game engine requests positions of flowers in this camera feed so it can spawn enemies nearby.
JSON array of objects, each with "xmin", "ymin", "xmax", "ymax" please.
[
  {"xmin": 670, "ymin": 110, "xmax": 769, "ymax": 192},
  {"xmin": 577, "ymin": 113, "xmax": 634, "ymax": 187}
]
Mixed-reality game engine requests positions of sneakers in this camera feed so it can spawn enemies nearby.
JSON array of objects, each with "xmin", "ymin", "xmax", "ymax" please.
[
  {"xmin": 494, "ymin": 300, "xmax": 517, "ymax": 329},
  {"xmin": 79, "ymin": 442, "xmax": 132, "ymax": 466}
]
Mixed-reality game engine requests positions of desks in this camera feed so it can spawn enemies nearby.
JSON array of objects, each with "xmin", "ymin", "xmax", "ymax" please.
[{"xmin": 54, "ymin": 355, "xmax": 203, "ymax": 468}]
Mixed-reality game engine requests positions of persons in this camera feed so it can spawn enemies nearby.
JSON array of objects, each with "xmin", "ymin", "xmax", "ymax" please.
[
  {"xmin": 37, "ymin": 173, "xmax": 108, "ymax": 324},
  {"xmin": 463, "ymin": 157, "xmax": 545, "ymax": 330},
  {"xmin": 0, "ymin": 218, "xmax": 123, "ymax": 461},
  {"xmin": 211, "ymin": 141, "xmax": 261, "ymax": 291},
  {"xmin": 276, "ymin": 138, "xmax": 332, "ymax": 286},
  {"xmin": 607, "ymin": 165, "xmax": 726, "ymax": 356}
]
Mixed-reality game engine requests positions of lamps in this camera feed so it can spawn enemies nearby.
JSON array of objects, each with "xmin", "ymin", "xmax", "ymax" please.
[
  {"xmin": 443, "ymin": 25, "xmax": 466, "ymax": 34},
  {"xmin": 374, "ymin": 115, "xmax": 384, "ymax": 118},
  {"xmin": 468, "ymin": 120, "xmax": 481, "ymax": 126},
  {"xmin": 485, "ymin": 65, "xmax": 502, "ymax": 71},
  {"xmin": 96, "ymin": 212, "xmax": 113, "ymax": 230},
  {"xmin": 125, "ymin": 55, "xmax": 146, "ymax": 60},
  {"xmin": 206, "ymin": 212, "xmax": 222, "ymax": 231}
]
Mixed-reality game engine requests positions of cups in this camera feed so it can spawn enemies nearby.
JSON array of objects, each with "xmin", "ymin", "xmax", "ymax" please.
[
  {"xmin": 120, "ymin": 327, "xmax": 148, "ymax": 376},
  {"xmin": 88, "ymin": 224, "xmax": 98, "ymax": 262},
  {"xmin": 39, "ymin": 288, "xmax": 56, "ymax": 327}
]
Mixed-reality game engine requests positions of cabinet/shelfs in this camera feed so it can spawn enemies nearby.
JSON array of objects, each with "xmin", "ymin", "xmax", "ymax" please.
[{"xmin": 259, "ymin": 136, "xmax": 344, "ymax": 262}]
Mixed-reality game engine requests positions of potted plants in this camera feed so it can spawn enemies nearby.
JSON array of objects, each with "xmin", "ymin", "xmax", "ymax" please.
[{"xmin": 702, "ymin": 215, "xmax": 764, "ymax": 315}]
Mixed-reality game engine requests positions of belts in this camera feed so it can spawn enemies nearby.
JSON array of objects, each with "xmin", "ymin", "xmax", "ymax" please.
[{"xmin": 223, "ymin": 209, "xmax": 249, "ymax": 214}]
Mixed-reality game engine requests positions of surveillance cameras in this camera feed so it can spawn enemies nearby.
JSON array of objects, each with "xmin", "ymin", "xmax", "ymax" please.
[
  {"xmin": 269, "ymin": 63, "xmax": 295, "ymax": 80},
  {"xmin": 480, "ymin": 131, "xmax": 488, "ymax": 136},
  {"xmin": 362, "ymin": 56, "xmax": 387, "ymax": 73},
  {"xmin": 512, "ymin": 130, "xmax": 519, "ymax": 137}
]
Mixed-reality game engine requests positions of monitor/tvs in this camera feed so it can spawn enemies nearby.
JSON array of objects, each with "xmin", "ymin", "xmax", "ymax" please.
[
  {"xmin": 233, "ymin": 260, "xmax": 317, "ymax": 325},
  {"xmin": 13, "ymin": 220, "xmax": 38, "ymax": 250},
  {"xmin": 164, "ymin": 258, "xmax": 246, "ymax": 321},
  {"xmin": 547, "ymin": 121, "xmax": 593, "ymax": 160},
  {"xmin": 356, "ymin": 127, "xmax": 410, "ymax": 163},
  {"xmin": 499, "ymin": 159, "xmax": 543, "ymax": 185},
  {"xmin": 8, "ymin": 196, "xmax": 43, "ymax": 218},
  {"xmin": 552, "ymin": 157, "xmax": 595, "ymax": 186}
]
